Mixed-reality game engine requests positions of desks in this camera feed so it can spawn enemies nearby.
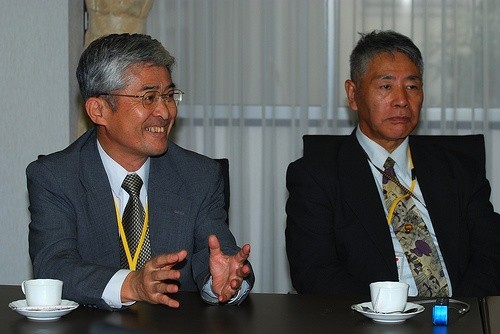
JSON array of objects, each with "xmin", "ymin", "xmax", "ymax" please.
[{"xmin": 0, "ymin": 285, "xmax": 500, "ymax": 334}]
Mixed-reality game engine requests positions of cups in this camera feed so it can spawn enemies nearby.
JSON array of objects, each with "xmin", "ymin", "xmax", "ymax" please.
[
  {"xmin": 369, "ymin": 281, "xmax": 409, "ymax": 314},
  {"xmin": 21, "ymin": 278, "xmax": 64, "ymax": 311}
]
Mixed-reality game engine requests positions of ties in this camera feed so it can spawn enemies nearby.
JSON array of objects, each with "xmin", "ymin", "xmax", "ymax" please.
[
  {"xmin": 383, "ymin": 157, "xmax": 450, "ymax": 297},
  {"xmin": 118, "ymin": 173, "xmax": 152, "ymax": 271}
]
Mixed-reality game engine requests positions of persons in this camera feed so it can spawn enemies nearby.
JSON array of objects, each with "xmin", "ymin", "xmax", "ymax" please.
[
  {"xmin": 26, "ymin": 33, "xmax": 255, "ymax": 311},
  {"xmin": 284, "ymin": 29, "xmax": 500, "ymax": 298}
]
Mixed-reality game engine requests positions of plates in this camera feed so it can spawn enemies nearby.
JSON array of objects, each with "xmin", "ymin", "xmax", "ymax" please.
[
  {"xmin": 351, "ymin": 301, "xmax": 426, "ymax": 322},
  {"xmin": 8, "ymin": 299, "xmax": 79, "ymax": 320}
]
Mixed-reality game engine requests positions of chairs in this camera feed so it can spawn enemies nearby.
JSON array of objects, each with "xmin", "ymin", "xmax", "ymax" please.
[{"xmin": 303, "ymin": 132, "xmax": 487, "ymax": 173}]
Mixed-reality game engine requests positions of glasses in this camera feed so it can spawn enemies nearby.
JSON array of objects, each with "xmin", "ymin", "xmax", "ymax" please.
[{"xmin": 95, "ymin": 89, "xmax": 184, "ymax": 109}]
